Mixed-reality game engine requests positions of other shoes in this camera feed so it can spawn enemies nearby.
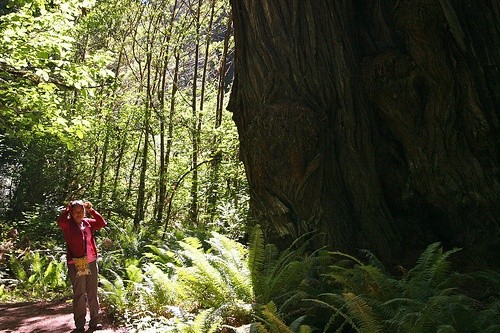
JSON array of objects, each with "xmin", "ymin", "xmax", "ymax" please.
[
  {"xmin": 90, "ymin": 324, "xmax": 102, "ymax": 330},
  {"xmin": 70, "ymin": 329, "xmax": 85, "ymax": 333}
]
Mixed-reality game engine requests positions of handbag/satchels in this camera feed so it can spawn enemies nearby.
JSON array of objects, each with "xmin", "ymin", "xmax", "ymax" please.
[{"xmin": 72, "ymin": 254, "xmax": 92, "ymax": 278}]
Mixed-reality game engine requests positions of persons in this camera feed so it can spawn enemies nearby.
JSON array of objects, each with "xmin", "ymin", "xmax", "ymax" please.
[{"xmin": 58, "ymin": 199, "xmax": 108, "ymax": 332}]
